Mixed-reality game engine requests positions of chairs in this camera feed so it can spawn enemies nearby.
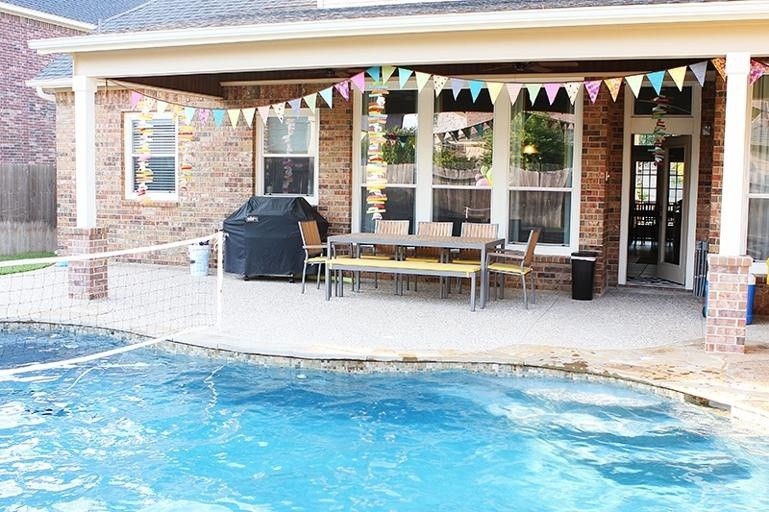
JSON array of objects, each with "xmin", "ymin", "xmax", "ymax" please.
[
  {"xmin": 297, "ymin": 218, "xmax": 544, "ymax": 311},
  {"xmin": 633, "ymin": 201, "xmax": 680, "ymax": 247},
  {"xmin": 465, "ymin": 206, "xmax": 490, "ymax": 224}
]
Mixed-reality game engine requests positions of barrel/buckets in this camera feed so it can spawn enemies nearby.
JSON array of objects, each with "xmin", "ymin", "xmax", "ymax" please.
[
  {"xmin": 745, "ymin": 272, "xmax": 757, "ymax": 324},
  {"xmin": 188, "ymin": 245, "xmax": 210, "ymax": 276}
]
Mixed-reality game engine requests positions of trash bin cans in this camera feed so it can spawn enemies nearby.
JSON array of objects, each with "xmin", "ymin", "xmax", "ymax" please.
[
  {"xmin": 570, "ymin": 252, "xmax": 598, "ymax": 300},
  {"xmin": 703, "ymin": 274, "xmax": 756, "ymax": 326}
]
